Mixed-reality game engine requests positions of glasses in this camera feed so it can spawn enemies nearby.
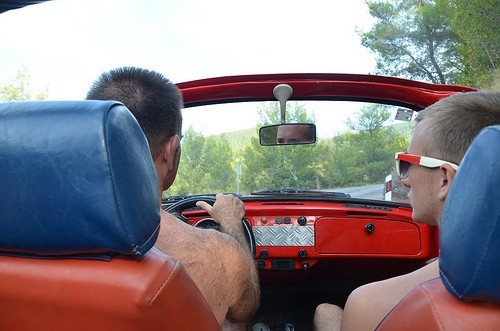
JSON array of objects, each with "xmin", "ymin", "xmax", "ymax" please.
[{"xmin": 395, "ymin": 152, "xmax": 460, "ymax": 179}]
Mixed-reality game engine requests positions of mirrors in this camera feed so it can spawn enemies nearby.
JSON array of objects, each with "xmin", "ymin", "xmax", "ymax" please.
[{"xmin": 259, "ymin": 123, "xmax": 316, "ymax": 146}]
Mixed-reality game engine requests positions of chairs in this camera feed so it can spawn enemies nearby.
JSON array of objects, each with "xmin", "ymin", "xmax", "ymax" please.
[{"xmin": 0, "ymin": 101, "xmax": 500, "ymax": 331}]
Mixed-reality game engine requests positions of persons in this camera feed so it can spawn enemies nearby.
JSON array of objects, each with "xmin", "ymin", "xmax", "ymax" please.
[
  {"xmin": 313, "ymin": 88, "xmax": 500, "ymax": 331},
  {"xmin": 85, "ymin": 68, "xmax": 271, "ymax": 331},
  {"xmin": 277, "ymin": 124, "xmax": 313, "ymax": 144}
]
